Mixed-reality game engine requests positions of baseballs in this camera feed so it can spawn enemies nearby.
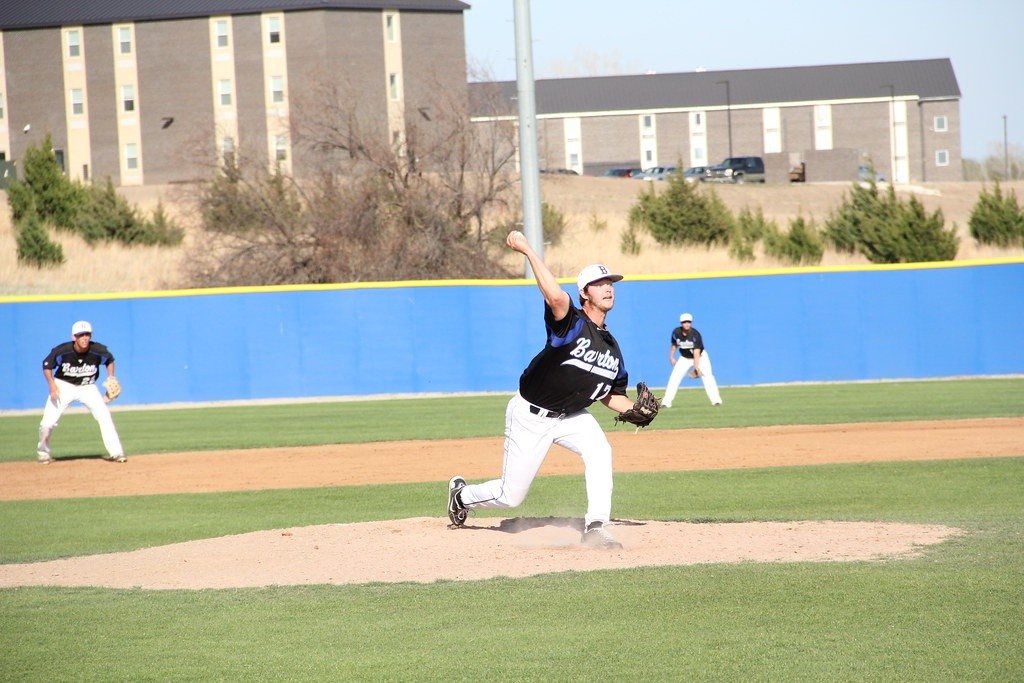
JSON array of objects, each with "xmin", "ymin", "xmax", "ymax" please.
[{"xmin": 513, "ymin": 231, "xmax": 523, "ymax": 239}]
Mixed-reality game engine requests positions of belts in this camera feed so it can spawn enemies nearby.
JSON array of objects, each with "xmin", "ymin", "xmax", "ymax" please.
[{"xmin": 530, "ymin": 404, "xmax": 571, "ymax": 422}]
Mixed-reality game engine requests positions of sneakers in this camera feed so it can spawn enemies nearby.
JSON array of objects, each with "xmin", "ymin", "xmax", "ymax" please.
[
  {"xmin": 581, "ymin": 525, "xmax": 622, "ymax": 551},
  {"xmin": 446, "ymin": 475, "xmax": 468, "ymax": 527}
]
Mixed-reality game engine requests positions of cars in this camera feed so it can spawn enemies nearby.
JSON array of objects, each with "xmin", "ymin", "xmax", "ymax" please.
[
  {"xmin": 682, "ymin": 167, "xmax": 708, "ymax": 183},
  {"xmin": 856, "ymin": 166, "xmax": 887, "ymax": 182},
  {"xmin": 605, "ymin": 168, "xmax": 642, "ymax": 179},
  {"xmin": 632, "ymin": 166, "xmax": 676, "ymax": 181}
]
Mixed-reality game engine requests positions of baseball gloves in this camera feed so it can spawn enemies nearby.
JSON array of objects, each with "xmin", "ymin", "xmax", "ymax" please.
[
  {"xmin": 102, "ymin": 376, "xmax": 121, "ymax": 404},
  {"xmin": 614, "ymin": 381, "xmax": 664, "ymax": 435},
  {"xmin": 689, "ymin": 368, "xmax": 704, "ymax": 379}
]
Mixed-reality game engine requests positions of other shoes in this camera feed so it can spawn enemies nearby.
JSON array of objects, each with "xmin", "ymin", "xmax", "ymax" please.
[
  {"xmin": 114, "ymin": 456, "xmax": 127, "ymax": 462},
  {"xmin": 716, "ymin": 402, "xmax": 720, "ymax": 407},
  {"xmin": 40, "ymin": 459, "xmax": 52, "ymax": 466},
  {"xmin": 660, "ymin": 404, "xmax": 669, "ymax": 408}
]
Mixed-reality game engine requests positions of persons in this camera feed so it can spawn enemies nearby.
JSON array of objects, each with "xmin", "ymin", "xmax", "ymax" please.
[
  {"xmin": 447, "ymin": 230, "xmax": 661, "ymax": 549},
  {"xmin": 660, "ymin": 313, "xmax": 722, "ymax": 407},
  {"xmin": 37, "ymin": 321, "xmax": 126, "ymax": 465}
]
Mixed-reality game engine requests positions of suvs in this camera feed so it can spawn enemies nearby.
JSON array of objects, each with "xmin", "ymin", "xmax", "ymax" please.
[{"xmin": 702, "ymin": 156, "xmax": 766, "ymax": 183}]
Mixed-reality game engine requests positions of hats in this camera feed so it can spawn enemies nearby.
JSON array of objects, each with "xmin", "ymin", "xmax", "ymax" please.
[
  {"xmin": 577, "ymin": 264, "xmax": 624, "ymax": 292},
  {"xmin": 679, "ymin": 313, "xmax": 694, "ymax": 324},
  {"xmin": 72, "ymin": 320, "xmax": 94, "ymax": 339}
]
